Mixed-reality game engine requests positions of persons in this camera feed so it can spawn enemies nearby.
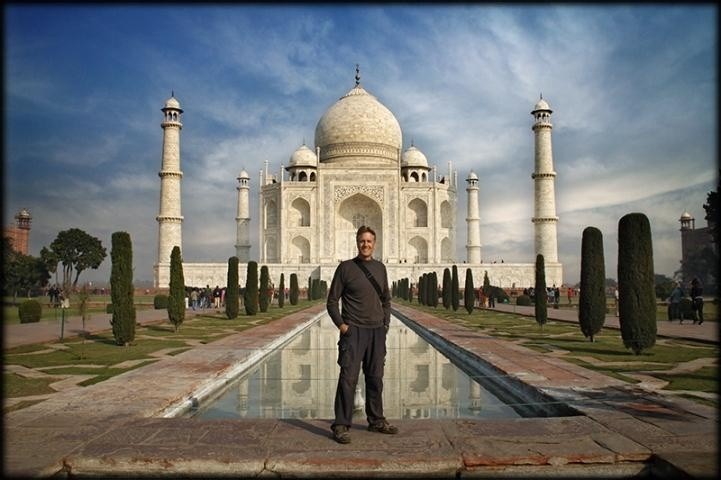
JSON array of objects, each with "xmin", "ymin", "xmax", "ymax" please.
[
  {"xmin": 567, "ymin": 288, "xmax": 572, "ymax": 304},
  {"xmin": 547, "ymin": 288, "xmax": 560, "ymax": 309},
  {"xmin": 49, "ymin": 287, "xmax": 60, "ymax": 303},
  {"xmin": 691, "ymin": 278, "xmax": 704, "ymax": 325},
  {"xmin": 670, "ymin": 282, "xmax": 684, "ymax": 325},
  {"xmin": 326, "ymin": 226, "xmax": 398, "ymax": 443},
  {"xmin": 190, "ymin": 285, "xmax": 225, "ymax": 312},
  {"xmin": 488, "ymin": 297, "xmax": 495, "ymax": 308},
  {"xmin": 614, "ymin": 284, "xmax": 619, "ymax": 317},
  {"xmin": 479, "ymin": 286, "xmax": 483, "ymax": 306},
  {"xmin": 285, "ymin": 288, "xmax": 288, "ymax": 299}
]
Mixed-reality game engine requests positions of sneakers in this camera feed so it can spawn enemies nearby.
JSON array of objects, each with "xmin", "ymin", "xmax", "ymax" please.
[
  {"xmin": 334, "ymin": 425, "xmax": 351, "ymax": 443},
  {"xmin": 368, "ymin": 421, "xmax": 398, "ymax": 434}
]
